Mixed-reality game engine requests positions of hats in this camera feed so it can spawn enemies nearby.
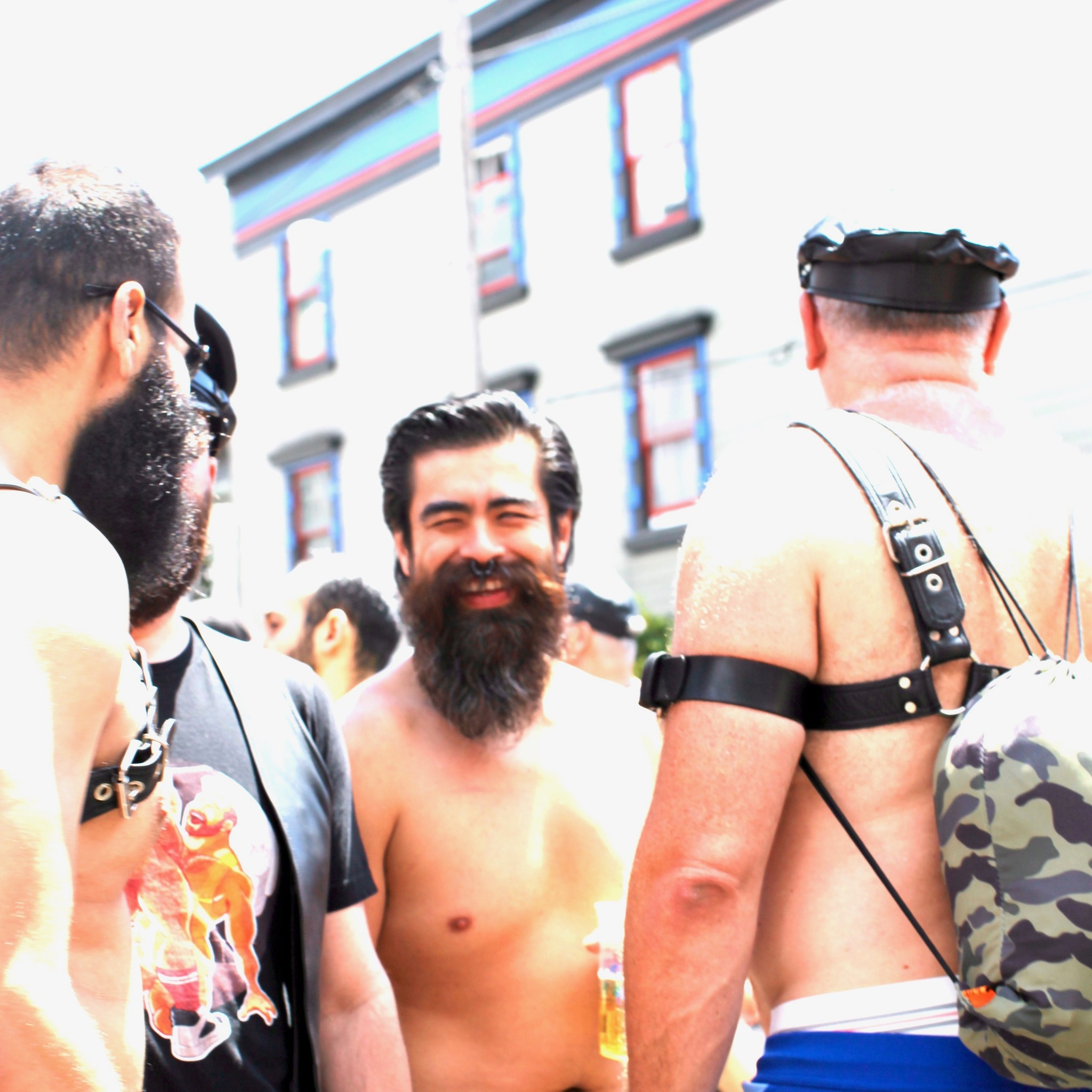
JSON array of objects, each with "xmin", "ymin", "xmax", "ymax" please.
[
  {"xmin": 796, "ymin": 214, "xmax": 1019, "ymax": 315},
  {"xmin": 180, "ymin": 299, "xmax": 239, "ymax": 456},
  {"xmin": 557, "ymin": 564, "xmax": 646, "ymax": 640}
]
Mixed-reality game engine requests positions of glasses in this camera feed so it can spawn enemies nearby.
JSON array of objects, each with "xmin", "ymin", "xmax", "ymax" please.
[{"xmin": 82, "ymin": 283, "xmax": 209, "ymax": 383}]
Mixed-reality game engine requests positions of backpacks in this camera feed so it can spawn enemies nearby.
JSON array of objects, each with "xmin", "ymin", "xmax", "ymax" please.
[{"xmin": 794, "ymin": 404, "xmax": 1092, "ymax": 1092}]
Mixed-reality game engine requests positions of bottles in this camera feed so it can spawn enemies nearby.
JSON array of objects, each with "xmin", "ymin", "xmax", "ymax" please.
[{"xmin": 593, "ymin": 898, "xmax": 626, "ymax": 1063}]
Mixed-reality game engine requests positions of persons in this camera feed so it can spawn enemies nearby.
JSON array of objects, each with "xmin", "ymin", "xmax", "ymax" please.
[{"xmin": 0, "ymin": 158, "xmax": 1091, "ymax": 1092}]
